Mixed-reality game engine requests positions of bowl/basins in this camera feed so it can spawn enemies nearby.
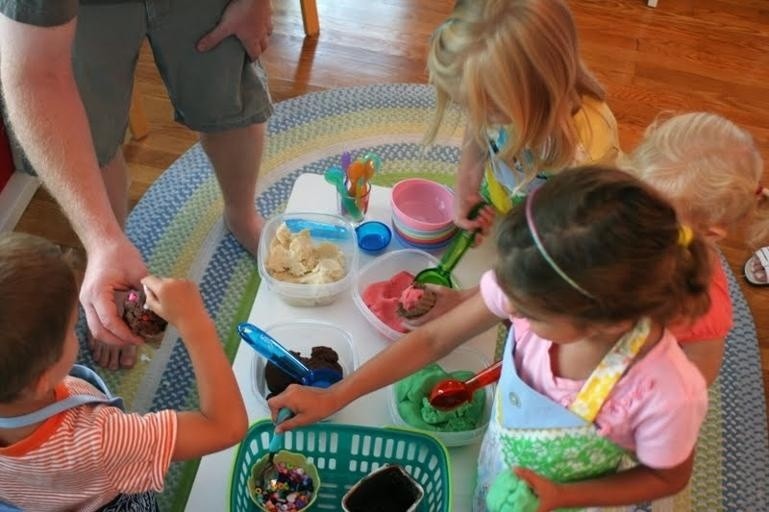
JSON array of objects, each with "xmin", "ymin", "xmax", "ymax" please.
[
  {"xmin": 388, "ymin": 181, "xmax": 462, "ymax": 251},
  {"xmin": 245, "ymin": 451, "xmax": 321, "ymax": 510}
]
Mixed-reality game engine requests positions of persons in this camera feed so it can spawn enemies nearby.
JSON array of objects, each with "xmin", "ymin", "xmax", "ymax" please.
[
  {"xmin": 266, "ymin": 166, "xmax": 711, "ymax": 510},
  {"xmin": 1, "ymin": 231, "xmax": 248, "ymax": 512},
  {"xmin": 430, "ymin": 1, "xmax": 619, "ymax": 235},
  {"xmin": 623, "ymin": 112, "xmax": 762, "ymax": 389},
  {"xmin": 0, "ymin": 1, "xmax": 278, "ymax": 372}
]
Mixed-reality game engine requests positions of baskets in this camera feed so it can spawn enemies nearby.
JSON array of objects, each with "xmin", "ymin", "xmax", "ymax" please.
[{"xmin": 226, "ymin": 418, "xmax": 453, "ymax": 512}]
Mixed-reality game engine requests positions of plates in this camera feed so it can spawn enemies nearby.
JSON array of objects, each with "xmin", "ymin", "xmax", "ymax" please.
[
  {"xmin": 355, "ymin": 223, "xmax": 391, "ymax": 252},
  {"xmin": 337, "ymin": 464, "xmax": 426, "ymax": 512}
]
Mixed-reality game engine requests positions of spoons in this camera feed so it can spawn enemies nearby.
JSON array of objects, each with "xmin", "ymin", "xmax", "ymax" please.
[{"xmin": 325, "ymin": 151, "xmax": 379, "ymax": 224}]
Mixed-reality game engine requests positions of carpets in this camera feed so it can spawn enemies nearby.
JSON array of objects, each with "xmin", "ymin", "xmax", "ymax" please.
[{"xmin": 43, "ymin": 82, "xmax": 766, "ymax": 511}]
